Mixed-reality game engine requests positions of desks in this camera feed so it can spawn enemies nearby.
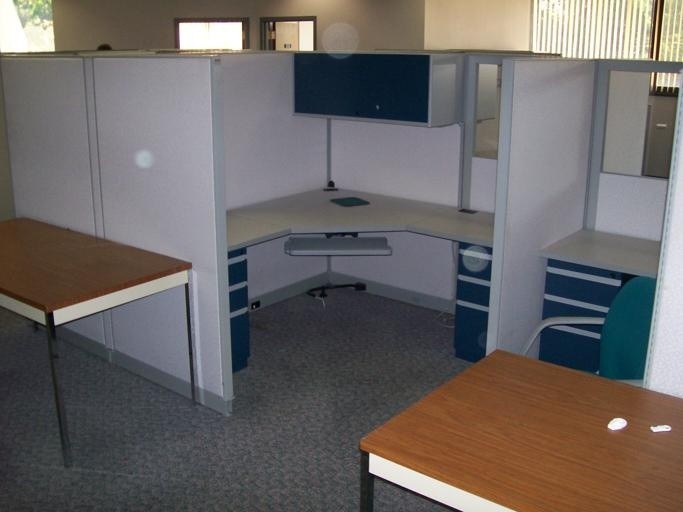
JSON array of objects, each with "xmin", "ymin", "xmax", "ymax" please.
[
  {"xmin": 538, "ymin": 229, "xmax": 659, "ymax": 280},
  {"xmin": 357, "ymin": 349, "xmax": 682, "ymax": 511},
  {"xmin": 0, "ymin": 217, "xmax": 196, "ymax": 469},
  {"xmin": 224, "ymin": 188, "xmax": 494, "ymax": 253}
]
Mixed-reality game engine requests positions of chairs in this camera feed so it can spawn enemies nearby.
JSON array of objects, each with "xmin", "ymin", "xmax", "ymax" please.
[{"xmin": 597, "ymin": 277, "xmax": 656, "ymax": 389}]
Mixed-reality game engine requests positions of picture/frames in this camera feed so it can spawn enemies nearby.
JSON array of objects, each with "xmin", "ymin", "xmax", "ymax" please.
[{"xmin": 453, "ymin": 241, "xmax": 493, "ymax": 364}]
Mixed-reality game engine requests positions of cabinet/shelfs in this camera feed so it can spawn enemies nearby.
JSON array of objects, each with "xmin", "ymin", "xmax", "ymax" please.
[
  {"xmin": 538, "ymin": 258, "xmax": 621, "ymax": 377},
  {"xmin": 226, "ymin": 248, "xmax": 250, "ymax": 374}
]
